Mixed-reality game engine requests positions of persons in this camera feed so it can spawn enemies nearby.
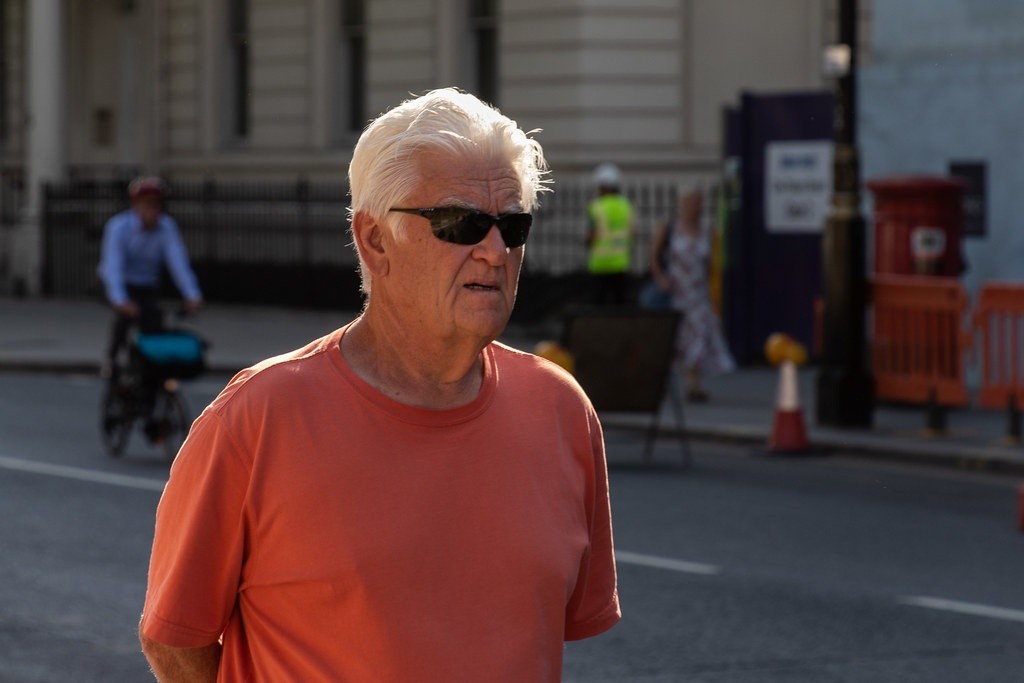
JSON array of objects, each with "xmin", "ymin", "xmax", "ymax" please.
[
  {"xmin": 138, "ymin": 88, "xmax": 621, "ymax": 683},
  {"xmin": 95, "ymin": 161, "xmax": 202, "ymax": 434},
  {"xmin": 584, "ymin": 164, "xmax": 638, "ymax": 273},
  {"xmin": 650, "ymin": 189, "xmax": 734, "ymax": 403}
]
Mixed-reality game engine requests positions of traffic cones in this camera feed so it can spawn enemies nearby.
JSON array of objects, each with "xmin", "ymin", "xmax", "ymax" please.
[{"xmin": 754, "ymin": 359, "xmax": 819, "ymax": 458}]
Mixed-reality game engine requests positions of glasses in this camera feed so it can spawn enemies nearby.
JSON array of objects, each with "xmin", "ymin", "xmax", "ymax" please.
[{"xmin": 390, "ymin": 206, "xmax": 532, "ymax": 248}]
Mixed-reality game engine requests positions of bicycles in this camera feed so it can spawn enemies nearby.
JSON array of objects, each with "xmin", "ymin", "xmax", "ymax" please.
[{"xmin": 94, "ymin": 300, "xmax": 213, "ymax": 477}]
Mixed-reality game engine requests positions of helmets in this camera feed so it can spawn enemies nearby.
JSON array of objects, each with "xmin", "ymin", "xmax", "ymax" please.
[{"xmin": 592, "ymin": 163, "xmax": 622, "ymax": 188}]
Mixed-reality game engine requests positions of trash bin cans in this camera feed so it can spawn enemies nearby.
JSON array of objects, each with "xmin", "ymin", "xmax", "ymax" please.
[{"xmin": 544, "ymin": 271, "xmax": 647, "ymax": 415}]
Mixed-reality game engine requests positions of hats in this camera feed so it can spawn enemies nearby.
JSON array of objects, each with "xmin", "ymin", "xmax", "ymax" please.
[{"xmin": 129, "ymin": 162, "xmax": 168, "ymax": 196}]
